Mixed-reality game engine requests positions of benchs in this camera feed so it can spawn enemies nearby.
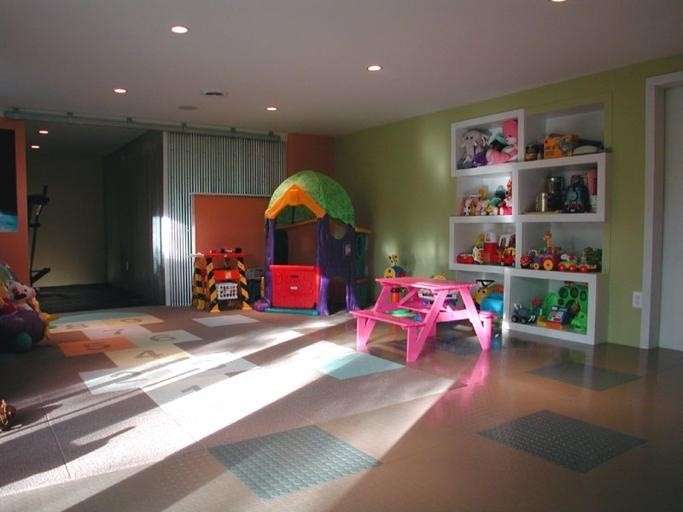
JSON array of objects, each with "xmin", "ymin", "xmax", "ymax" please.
[
  {"xmin": 346, "ymin": 305, "xmax": 426, "ymax": 363},
  {"xmin": 419, "ymin": 296, "xmax": 494, "ymax": 352}
]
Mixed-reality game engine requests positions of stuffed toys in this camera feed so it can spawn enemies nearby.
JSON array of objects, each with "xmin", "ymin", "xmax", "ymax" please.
[
  {"xmin": 457, "ymin": 120, "xmax": 518, "ymax": 170},
  {"xmin": 459, "ymin": 180, "xmax": 512, "ymax": 216},
  {"xmin": 0, "ymin": 259, "xmax": 60, "ymax": 351}
]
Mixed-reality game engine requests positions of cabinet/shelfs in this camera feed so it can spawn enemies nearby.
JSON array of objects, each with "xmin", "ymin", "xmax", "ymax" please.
[
  {"xmin": 448, "ymin": 93, "xmax": 621, "ymax": 346},
  {"xmin": 191, "ymin": 251, "xmax": 253, "ymax": 312}
]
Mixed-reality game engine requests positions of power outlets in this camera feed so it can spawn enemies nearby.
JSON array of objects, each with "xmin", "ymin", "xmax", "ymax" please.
[{"xmin": 632, "ymin": 290, "xmax": 641, "ymax": 309}]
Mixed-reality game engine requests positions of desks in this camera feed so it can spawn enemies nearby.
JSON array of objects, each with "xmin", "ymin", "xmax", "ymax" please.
[{"xmin": 372, "ymin": 276, "xmax": 482, "ymax": 363}]
